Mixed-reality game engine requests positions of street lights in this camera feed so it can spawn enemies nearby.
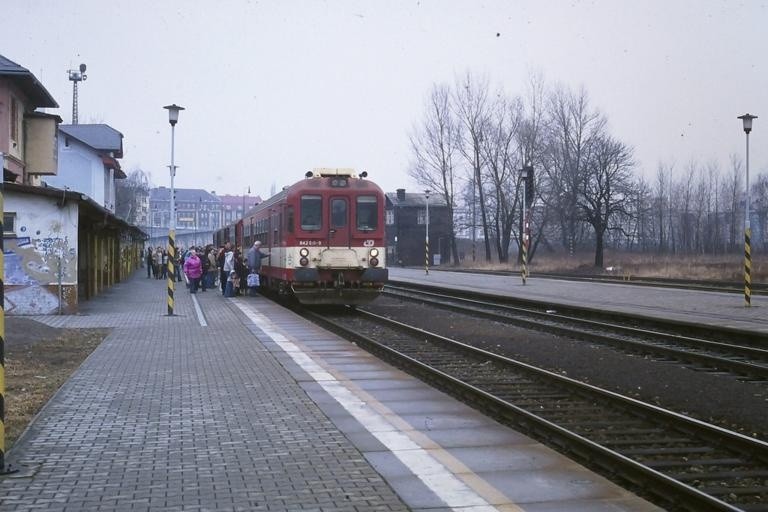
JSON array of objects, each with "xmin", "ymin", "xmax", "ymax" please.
[
  {"xmin": 422, "ymin": 187, "xmax": 431, "ymax": 275},
  {"xmin": 162, "ymin": 103, "xmax": 185, "ymax": 316},
  {"xmin": 519, "ymin": 166, "xmax": 529, "ymax": 287},
  {"xmin": 737, "ymin": 113, "xmax": 758, "ymax": 311}
]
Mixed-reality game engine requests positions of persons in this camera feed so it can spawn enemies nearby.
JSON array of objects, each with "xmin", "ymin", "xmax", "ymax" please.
[{"xmin": 140, "ymin": 239, "xmax": 271, "ymax": 298}]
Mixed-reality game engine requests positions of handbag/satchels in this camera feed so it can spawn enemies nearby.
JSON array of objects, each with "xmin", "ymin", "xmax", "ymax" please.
[{"xmin": 247, "ymin": 274, "xmax": 260, "ymax": 287}]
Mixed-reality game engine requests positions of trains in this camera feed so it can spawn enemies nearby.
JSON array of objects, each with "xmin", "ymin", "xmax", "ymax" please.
[{"xmin": 212, "ymin": 167, "xmax": 388, "ymax": 305}]
